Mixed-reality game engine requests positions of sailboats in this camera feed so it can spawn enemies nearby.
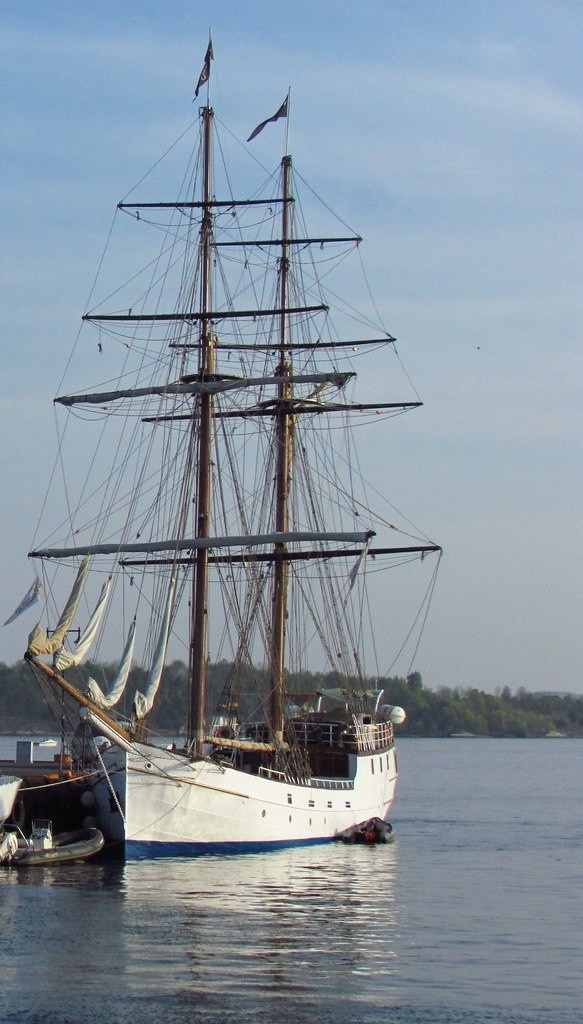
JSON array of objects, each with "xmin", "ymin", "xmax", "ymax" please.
[{"xmin": 25, "ymin": 22, "xmax": 447, "ymax": 862}]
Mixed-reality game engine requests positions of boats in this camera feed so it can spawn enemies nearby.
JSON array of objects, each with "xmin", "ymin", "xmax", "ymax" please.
[
  {"xmin": 1, "ymin": 819, "xmax": 105, "ymax": 865},
  {"xmin": 32, "ymin": 738, "xmax": 59, "ymax": 747},
  {"xmin": 341, "ymin": 816, "xmax": 396, "ymax": 843}
]
